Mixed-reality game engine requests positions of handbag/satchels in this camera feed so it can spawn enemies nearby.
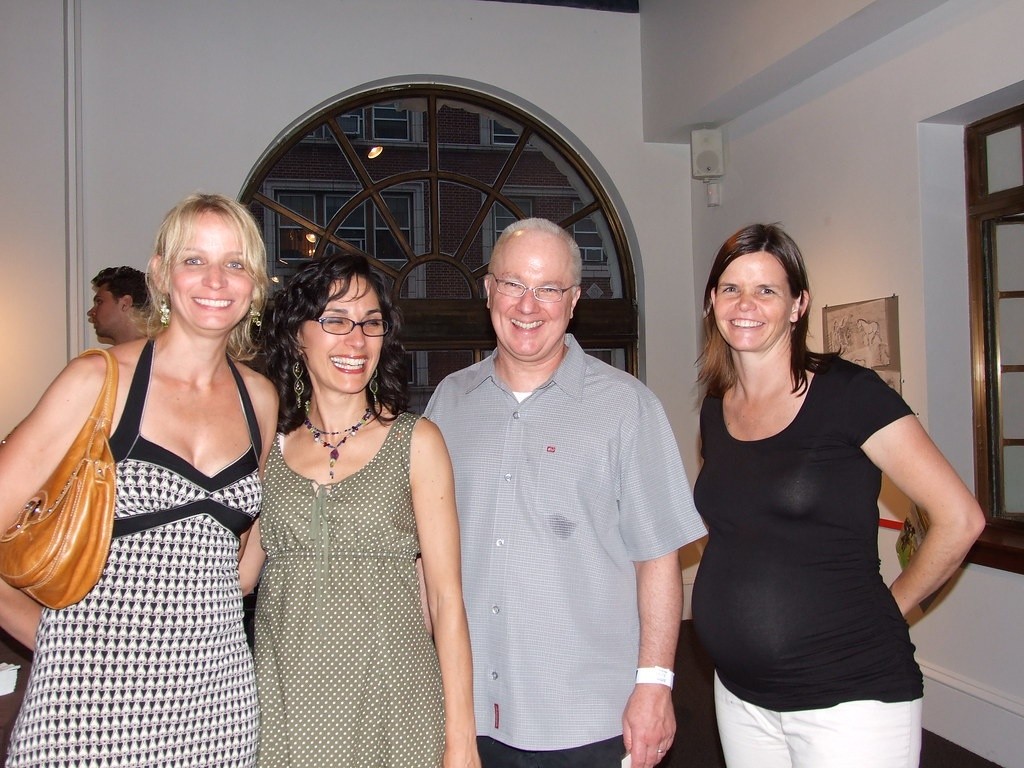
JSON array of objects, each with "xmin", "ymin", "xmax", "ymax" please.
[{"xmin": 0, "ymin": 347, "xmax": 120, "ymax": 609}]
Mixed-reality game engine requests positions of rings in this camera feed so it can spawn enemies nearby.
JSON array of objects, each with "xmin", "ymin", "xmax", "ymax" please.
[{"xmin": 658, "ymin": 750, "xmax": 663, "ymax": 753}]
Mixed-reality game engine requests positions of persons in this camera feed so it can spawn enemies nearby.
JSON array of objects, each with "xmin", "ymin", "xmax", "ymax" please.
[
  {"xmin": 87, "ymin": 266, "xmax": 150, "ymax": 345},
  {"xmin": 691, "ymin": 224, "xmax": 986, "ymax": 768},
  {"xmin": 0, "ymin": 196, "xmax": 278, "ymax": 768},
  {"xmin": 427, "ymin": 219, "xmax": 708, "ymax": 768},
  {"xmin": 237, "ymin": 254, "xmax": 480, "ymax": 768}
]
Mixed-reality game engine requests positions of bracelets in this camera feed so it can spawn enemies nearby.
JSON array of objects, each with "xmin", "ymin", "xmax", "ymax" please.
[{"xmin": 635, "ymin": 666, "xmax": 674, "ymax": 690}]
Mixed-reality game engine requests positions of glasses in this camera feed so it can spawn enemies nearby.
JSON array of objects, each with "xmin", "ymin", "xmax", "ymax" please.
[
  {"xmin": 490, "ymin": 272, "xmax": 577, "ymax": 303},
  {"xmin": 306, "ymin": 317, "xmax": 388, "ymax": 337}
]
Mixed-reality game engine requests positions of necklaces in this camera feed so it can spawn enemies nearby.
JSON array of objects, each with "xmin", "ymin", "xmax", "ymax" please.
[{"xmin": 304, "ymin": 408, "xmax": 372, "ymax": 480}]
